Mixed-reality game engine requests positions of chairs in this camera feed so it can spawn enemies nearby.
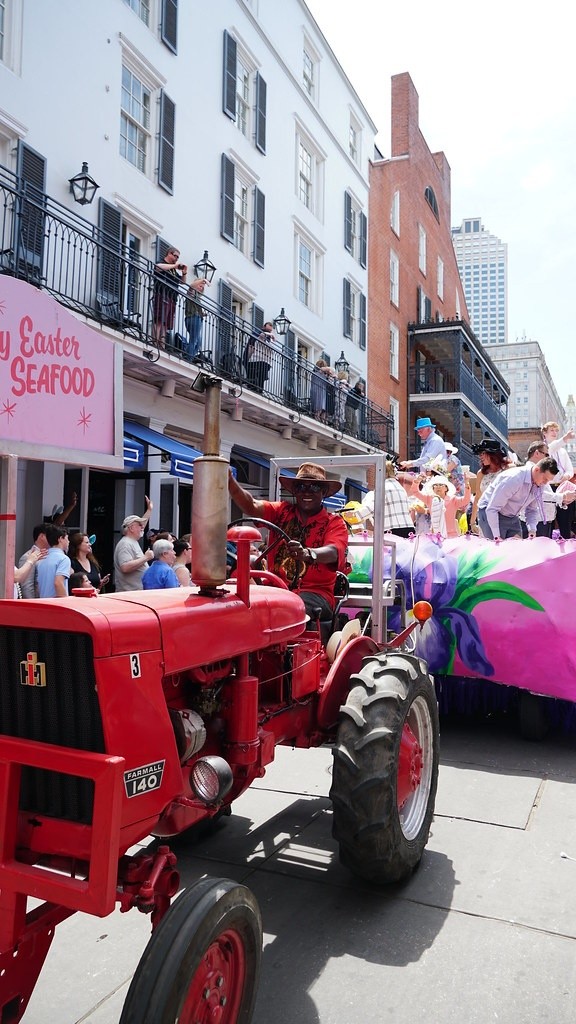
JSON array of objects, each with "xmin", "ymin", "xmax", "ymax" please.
[
  {"xmin": 0, "ymin": 248, "xmax": 48, "ymax": 292},
  {"xmin": 287, "ymin": 389, "xmax": 311, "ymax": 415},
  {"xmin": 342, "ymin": 422, "xmax": 388, "ymax": 448},
  {"xmin": 220, "ymin": 353, "xmax": 256, "ymax": 389},
  {"xmin": 175, "ymin": 332, "xmax": 214, "ymax": 373},
  {"xmin": 96, "ymin": 294, "xmax": 143, "ymax": 339},
  {"xmin": 417, "ymin": 378, "xmax": 434, "ymax": 394}
]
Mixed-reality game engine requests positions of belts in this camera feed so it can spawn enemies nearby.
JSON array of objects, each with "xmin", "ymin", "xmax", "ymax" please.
[{"xmin": 548, "ymin": 483, "xmax": 560, "ymax": 487}]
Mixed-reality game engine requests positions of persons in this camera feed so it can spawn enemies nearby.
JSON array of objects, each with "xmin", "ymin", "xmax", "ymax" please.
[
  {"xmin": 540, "ymin": 421, "xmax": 576, "ymax": 539},
  {"xmin": 68, "ymin": 532, "xmax": 104, "ymax": 594},
  {"xmin": 228, "ymin": 461, "xmax": 348, "ymax": 705},
  {"xmin": 226, "ymin": 535, "xmax": 266, "ymax": 584},
  {"xmin": 243, "ymin": 322, "xmax": 275, "ymax": 395},
  {"xmin": 141, "ymin": 528, "xmax": 196, "ymax": 590},
  {"xmin": 34, "ymin": 522, "xmax": 71, "ymax": 597},
  {"xmin": 398, "ymin": 418, "xmax": 559, "ymax": 542},
  {"xmin": 345, "ymin": 381, "xmax": 365, "ymax": 437},
  {"xmin": 14, "ymin": 546, "xmax": 49, "ymax": 599},
  {"xmin": 114, "ymin": 494, "xmax": 155, "ymax": 592},
  {"xmin": 184, "ymin": 277, "xmax": 212, "ymax": 365},
  {"xmin": 18, "ymin": 523, "xmax": 53, "ymax": 599},
  {"xmin": 153, "ymin": 246, "xmax": 188, "ymax": 350},
  {"xmin": 311, "ymin": 359, "xmax": 353, "ymax": 431},
  {"xmin": 344, "ymin": 460, "xmax": 415, "ymax": 538},
  {"xmin": 68, "ymin": 572, "xmax": 110, "ymax": 595}
]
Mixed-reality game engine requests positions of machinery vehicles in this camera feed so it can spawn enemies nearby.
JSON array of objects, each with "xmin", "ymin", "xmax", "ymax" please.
[{"xmin": 1, "ymin": 376, "xmax": 442, "ymax": 1023}]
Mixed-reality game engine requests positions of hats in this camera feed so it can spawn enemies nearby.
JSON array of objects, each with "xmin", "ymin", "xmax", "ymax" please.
[
  {"xmin": 279, "ymin": 462, "xmax": 343, "ymax": 498},
  {"xmin": 443, "ymin": 441, "xmax": 458, "ymax": 455},
  {"xmin": 327, "ymin": 618, "xmax": 361, "ymax": 664},
  {"xmin": 413, "ymin": 418, "xmax": 436, "ymax": 430},
  {"xmin": 123, "ymin": 515, "xmax": 148, "ymax": 527},
  {"xmin": 427, "ymin": 475, "xmax": 457, "ymax": 496},
  {"xmin": 472, "ymin": 439, "xmax": 507, "ymax": 457}
]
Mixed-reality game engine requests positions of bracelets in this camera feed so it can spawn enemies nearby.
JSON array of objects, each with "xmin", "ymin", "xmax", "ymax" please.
[
  {"xmin": 464, "ymin": 482, "xmax": 469, "ymax": 486},
  {"xmin": 27, "ymin": 560, "xmax": 34, "ymax": 567}
]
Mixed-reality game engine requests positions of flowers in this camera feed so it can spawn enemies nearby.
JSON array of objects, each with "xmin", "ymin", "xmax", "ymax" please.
[
  {"xmin": 471, "ymin": 442, "xmax": 486, "ymax": 456},
  {"xmin": 424, "ymin": 455, "xmax": 461, "ymax": 495}
]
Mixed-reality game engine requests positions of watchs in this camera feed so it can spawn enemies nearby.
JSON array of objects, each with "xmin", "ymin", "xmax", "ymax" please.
[
  {"xmin": 183, "ymin": 273, "xmax": 187, "ymax": 275},
  {"xmin": 306, "ymin": 547, "xmax": 318, "ymax": 565}
]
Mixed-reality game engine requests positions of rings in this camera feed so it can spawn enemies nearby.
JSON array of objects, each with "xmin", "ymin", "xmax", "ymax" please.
[{"xmin": 295, "ymin": 552, "xmax": 297, "ymax": 556}]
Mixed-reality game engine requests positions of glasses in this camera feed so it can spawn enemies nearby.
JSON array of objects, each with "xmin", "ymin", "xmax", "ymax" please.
[
  {"xmin": 533, "ymin": 449, "xmax": 550, "ymax": 458},
  {"xmin": 432, "ymin": 484, "xmax": 446, "ymax": 487},
  {"xmin": 170, "ymin": 251, "xmax": 179, "ymax": 260},
  {"xmin": 294, "ymin": 483, "xmax": 323, "ymax": 494}
]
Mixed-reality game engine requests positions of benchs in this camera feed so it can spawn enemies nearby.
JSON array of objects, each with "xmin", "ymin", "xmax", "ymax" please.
[{"xmin": 335, "ymin": 540, "xmax": 398, "ymax": 608}]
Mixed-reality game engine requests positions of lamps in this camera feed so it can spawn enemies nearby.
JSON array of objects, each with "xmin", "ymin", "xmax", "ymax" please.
[
  {"xmin": 334, "ymin": 351, "xmax": 349, "ymax": 372},
  {"xmin": 232, "ymin": 407, "xmax": 244, "ymax": 422},
  {"xmin": 308, "ymin": 436, "xmax": 318, "ymax": 450},
  {"xmin": 193, "ymin": 251, "xmax": 217, "ymax": 283},
  {"xmin": 282, "ymin": 426, "xmax": 292, "ymax": 440},
  {"xmin": 161, "ymin": 378, "xmax": 176, "ymax": 398},
  {"xmin": 333, "ymin": 445, "xmax": 341, "ymax": 456},
  {"xmin": 68, "ymin": 162, "xmax": 100, "ymax": 205},
  {"xmin": 272, "ymin": 307, "xmax": 292, "ymax": 334}
]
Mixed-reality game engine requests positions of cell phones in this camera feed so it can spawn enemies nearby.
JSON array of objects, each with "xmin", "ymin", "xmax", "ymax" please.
[
  {"xmin": 178, "ymin": 265, "xmax": 184, "ymax": 270},
  {"xmin": 105, "ymin": 574, "xmax": 111, "ymax": 579}
]
